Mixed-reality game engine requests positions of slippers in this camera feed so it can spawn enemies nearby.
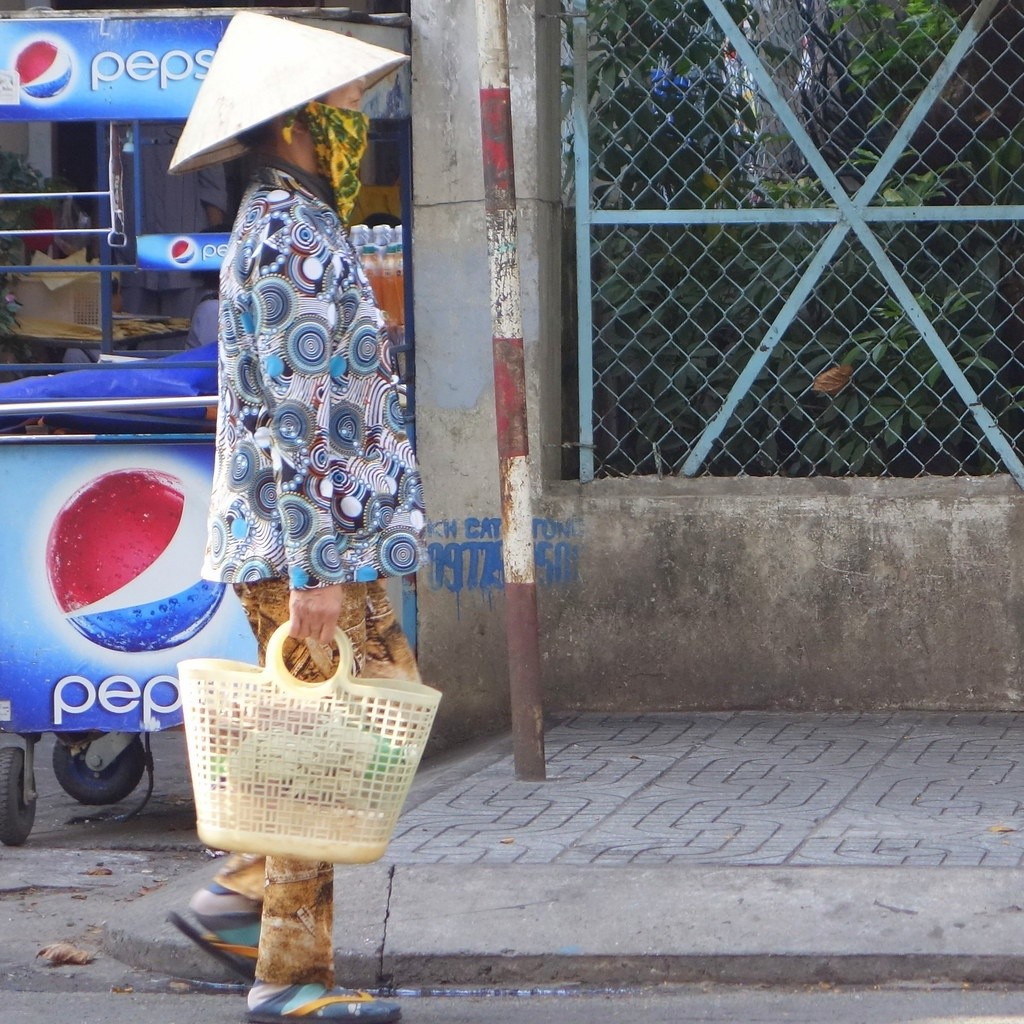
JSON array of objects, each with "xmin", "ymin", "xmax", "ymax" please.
[
  {"xmin": 167, "ymin": 903, "xmax": 257, "ymax": 977},
  {"xmin": 246, "ymin": 978, "xmax": 403, "ymax": 1024}
]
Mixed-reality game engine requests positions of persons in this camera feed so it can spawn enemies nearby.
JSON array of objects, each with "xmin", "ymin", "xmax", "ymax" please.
[{"xmin": 101, "ymin": 10, "xmax": 429, "ymax": 1024}]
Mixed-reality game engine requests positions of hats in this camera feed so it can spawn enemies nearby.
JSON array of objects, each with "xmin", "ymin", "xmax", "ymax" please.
[{"xmin": 167, "ymin": 9, "xmax": 411, "ymax": 177}]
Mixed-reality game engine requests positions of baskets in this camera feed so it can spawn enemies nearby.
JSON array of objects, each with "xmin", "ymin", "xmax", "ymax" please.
[
  {"xmin": 177, "ymin": 619, "xmax": 442, "ymax": 865},
  {"xmin": 14, "ymin": 278, "xmax": 101, "ymax": 327}
]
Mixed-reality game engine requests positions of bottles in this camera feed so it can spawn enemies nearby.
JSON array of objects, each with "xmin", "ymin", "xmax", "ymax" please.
[
  {"xmin": 350, "ymin": 225, "xmax": 405, "ymax": 325},
  {"xmin": 248, "ymin": 728, "xmax": 417, "ymax": 777}
]
